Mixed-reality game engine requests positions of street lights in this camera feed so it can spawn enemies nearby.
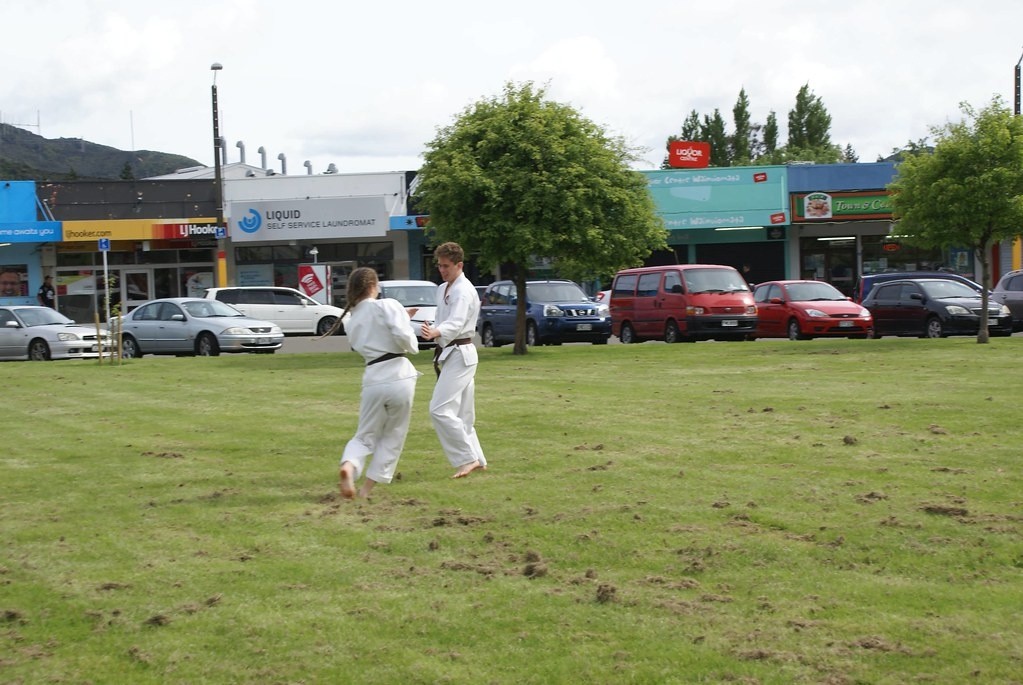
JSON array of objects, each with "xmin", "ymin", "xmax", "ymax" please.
[
  {"xmin": 211, "ymin": 64, "xmax": 228, "ymax": 286},
  {"xmin": 309, "ymin": 246, "xmax": 318, "ymax": 263}
]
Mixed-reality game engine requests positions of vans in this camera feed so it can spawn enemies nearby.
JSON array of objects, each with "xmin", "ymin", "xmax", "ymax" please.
[
  {"xmin": 853, "ymin": 272, "xmax": 993, "ymax": 304},
  {"xmin": 609, "ymin": 264, "xmax": 760, "ymax": 343}
]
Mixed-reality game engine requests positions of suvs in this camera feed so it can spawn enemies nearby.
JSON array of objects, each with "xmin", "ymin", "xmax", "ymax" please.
[
  {"xmin": 476, "ymin": 279, "xmax": 611, "ymax": 348},
  {"xmin": 993, "ymin": 270, "xmax": 1023, "ymax": 328}
]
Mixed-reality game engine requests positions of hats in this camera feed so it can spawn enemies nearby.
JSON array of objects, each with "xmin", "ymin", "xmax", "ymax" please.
[{"xmin": 44, "ymin": 275, "xmax": 53, "ymax": 279}]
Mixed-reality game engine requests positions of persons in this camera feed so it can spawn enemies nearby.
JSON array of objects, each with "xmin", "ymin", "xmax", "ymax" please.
[
  {"xmin": 38, "ymin": 276, "xmax": 56, "ymax": 309},
  {"xmin": 312, "ymin": 268, "xmax": 419, "ymax": 499},
  {"xmin": 4, "ymin": 283, "xmax": 21, "ymax": 296},
  {"xmin": 743, "ymin": 264, "xmax": 753, "ymax": 284},
  {"xmin": 410, "ymin": 243, "xmax": 487, "ymax": 479}
]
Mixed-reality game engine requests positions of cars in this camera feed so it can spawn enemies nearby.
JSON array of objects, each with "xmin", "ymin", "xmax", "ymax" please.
[
  {"xmin": 476, "ymin": 286, "xmax": 515, "ymax": 329},
  {"xmin": 377, "ymin": 281, "xmax": 439, "ymax": 350},
  {"xmin": 751, "ymin": 280, "xmax": 874, "ymax": 340},
  {"xmin": 597, "ymin": 288, "xmax": 612, "ymax": 307},
  {"xmin": 0, "ymin": 306, "xmax": 119, "ymax": 363},
  {"xmin": 203, "ymin": 286, "xmax": 352, "ymax": 337},
  {"xmin": 108, "ymin": 296, "xmax": 284, "ymax": 358},
  {"xmin": 861, "ymin": 278, "xmax": 1012, "ymax": 339}
]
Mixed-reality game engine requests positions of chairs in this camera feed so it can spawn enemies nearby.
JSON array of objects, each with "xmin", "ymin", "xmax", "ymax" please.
[{"xmin": 405, "ymin": 290, "xmax": 423, "ymax": 303}]
